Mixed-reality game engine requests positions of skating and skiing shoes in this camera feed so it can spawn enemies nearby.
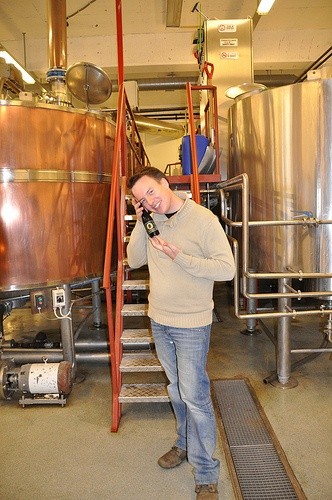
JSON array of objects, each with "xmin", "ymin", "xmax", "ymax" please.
[{"xmin": 158, "ymin": 446, "xmax": 187, "ymax": 469}]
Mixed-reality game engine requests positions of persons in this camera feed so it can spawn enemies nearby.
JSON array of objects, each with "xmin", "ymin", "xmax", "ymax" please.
[{"xmin": 126, "ymin": 166, "xmax": 236, "ymax": 500}]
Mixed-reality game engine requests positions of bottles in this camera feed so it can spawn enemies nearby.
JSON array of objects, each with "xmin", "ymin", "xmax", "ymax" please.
[{"xmin": 138, "ymin": 202, "xmax": 160, "ymax": 237}]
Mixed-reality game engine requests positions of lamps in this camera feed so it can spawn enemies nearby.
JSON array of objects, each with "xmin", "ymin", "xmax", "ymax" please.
[
  {"xmin": 0, "ymin": 33, "xmax": 37, "ymax": 84},
  {"xmin": 257, "ymin": 0, "xmax": 275, "ymax": 16}
]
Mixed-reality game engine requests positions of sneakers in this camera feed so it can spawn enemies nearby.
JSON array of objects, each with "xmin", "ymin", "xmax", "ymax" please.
[{"xmin": 195, "ymin": 482, "xmax": 219, "ymax": 500}]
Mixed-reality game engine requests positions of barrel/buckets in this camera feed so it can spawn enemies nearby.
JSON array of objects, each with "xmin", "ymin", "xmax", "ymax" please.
[{"xmin": 181, "ymin": 135, "xmax": 210, "ymax": 175}]
